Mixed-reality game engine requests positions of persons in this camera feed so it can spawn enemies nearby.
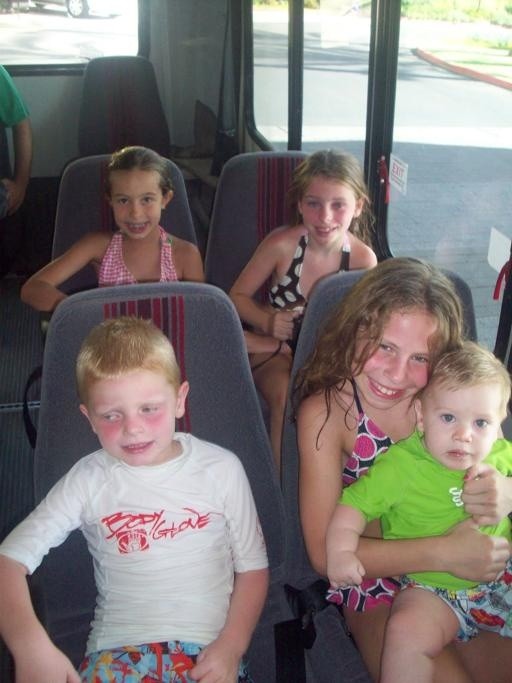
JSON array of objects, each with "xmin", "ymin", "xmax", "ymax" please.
[
  {"xmin": 325, "ymin": 350, "xmax": 511, "ymax": 683},
  {"xmin": 21, "ymin": 145, "xmax": 206, "ymax": 312},
  {"xmin": 227, "ymin": 149, "xmax": 381, "ymax": 490},
  {"xmin": 0, "ymin": 315, "xmax": 269, "ymax": 683},
  {"xmin": 2, "ymin": 65, "xmax": 33, "ymax": 280}
]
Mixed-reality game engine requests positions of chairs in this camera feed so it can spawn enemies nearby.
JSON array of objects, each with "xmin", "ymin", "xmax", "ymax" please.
[
  {"xmin": 32, "ymin": 282, "xmax": 317, "ymax": 683},
  {"xmin": 77, "ymin": 56, "xmax": 170, "ymax": 161},
  {"xmin": 53, "ymin": 155, "xmax": 197, "ymax": 296},
  {"xmin": 204, "ymin": 151, "xmax": 313, "ymax": 294}
]
